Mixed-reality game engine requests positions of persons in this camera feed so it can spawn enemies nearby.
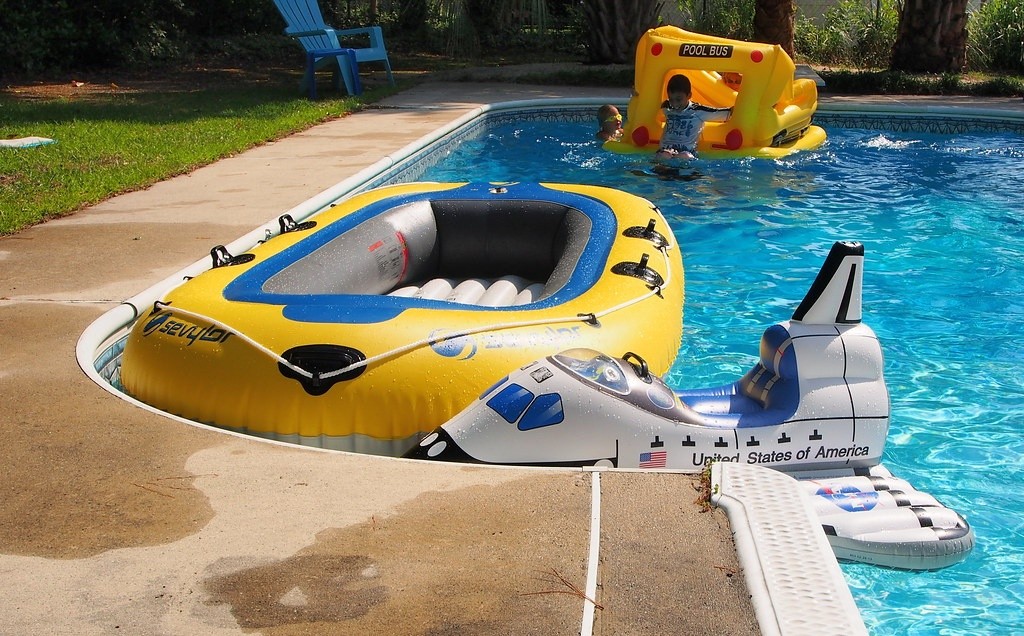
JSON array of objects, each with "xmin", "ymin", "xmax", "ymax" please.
[
  {"xmin": 657, "ymin": 74, "xmax": 734, "ymax": 161},
  {"xmin": 595, "ymin": 104, "xmax": 623, "ymax": 142},
  {"xmin": 722, "ymin": 72, "xmax": 743, "ymax": 91}
]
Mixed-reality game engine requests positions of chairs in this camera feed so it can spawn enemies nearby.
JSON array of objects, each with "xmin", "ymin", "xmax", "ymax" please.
[{"xmin": 273, "ymin": 0, "xmax": 396, "ymax": 99}]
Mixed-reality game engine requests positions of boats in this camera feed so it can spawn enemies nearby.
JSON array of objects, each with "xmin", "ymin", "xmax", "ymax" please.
[
  {"xmin": 120, "ymin": 181, "xmax": 685, "ymax": 457},
  {"xmin": 602, "ymin": 25, "xmax": 827, "ymax": 161}
]
